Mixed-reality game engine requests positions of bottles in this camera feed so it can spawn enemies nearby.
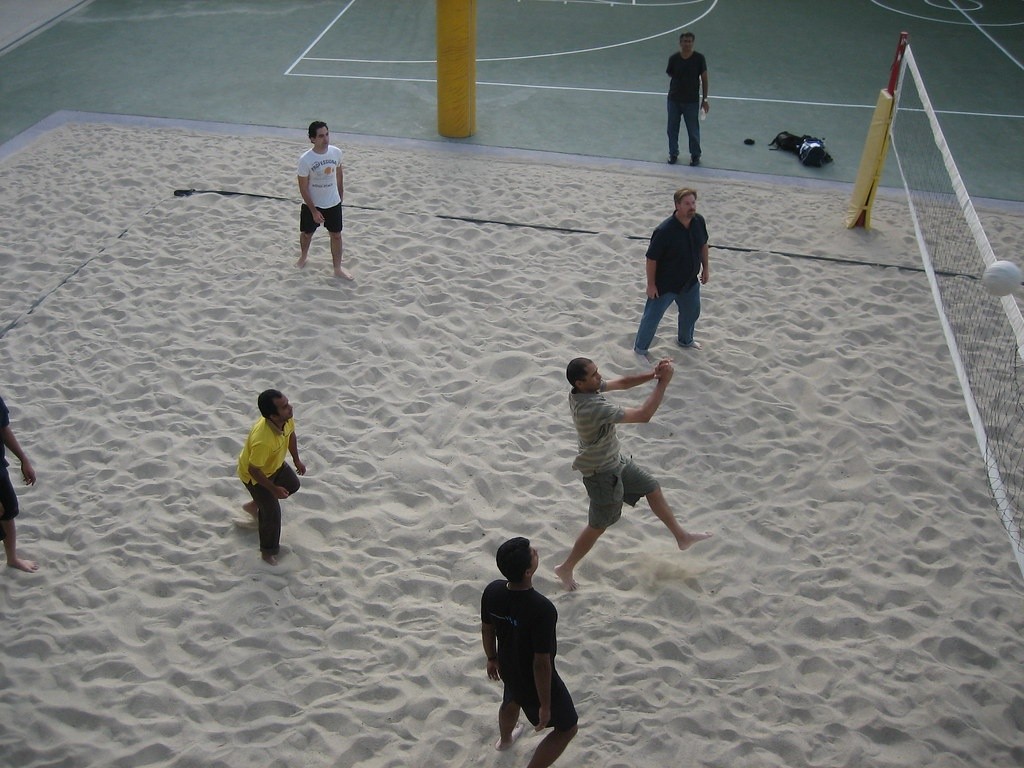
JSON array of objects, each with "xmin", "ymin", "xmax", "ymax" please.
[{"xmin": 700, "ymin": 108, "xmax": 705, "ymax": 120}]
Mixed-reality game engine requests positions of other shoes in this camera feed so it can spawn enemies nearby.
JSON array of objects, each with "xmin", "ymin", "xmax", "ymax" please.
[
  {"xmin": 668, "ymin": 152, "xmax": 677, "ymax": 163},
  {"xmin": 690, "ymin": 155, "xmax": 700, "ymax": 166}
]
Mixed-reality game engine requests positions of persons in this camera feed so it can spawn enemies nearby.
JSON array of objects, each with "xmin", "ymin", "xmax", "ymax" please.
[
  {"xmin": 294, "ymin": 121, "xmax": 355, "ymax": 281},
  {"xmin": 480, "ymin": 537, "xmax": 579, "ymax": 768},
  {"xmin": 666, "ymin": 32, "xmax": 710, "ymax": 166},
  {"xmin": 237, "ymin": 389, "xmax": 306, "ymax": 566},
  {"xmin": 0, "ymin": 396, "xmax": 40, "ymax": 574},
  {"xmin": 633, "ymin": 187, "xmax": 709, "ymax": 355},
  {"xmin": 554, "ymin": 358, "xmax": 711, "ymax": 591}
]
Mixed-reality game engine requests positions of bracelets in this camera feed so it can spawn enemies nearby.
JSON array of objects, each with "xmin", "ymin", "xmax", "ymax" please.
[
  {"xmin": 702, "ymin": 99, "xmax": 708, "ymax": 102},
  {"xmin": 488, "ymin": 656, "xmax": 497, "ymax": 660}
]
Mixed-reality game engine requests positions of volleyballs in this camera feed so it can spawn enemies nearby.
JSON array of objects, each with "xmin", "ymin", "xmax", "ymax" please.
[{"xmin": 982, "ymin": 259, "xmax": 1021, "ymax": 297}]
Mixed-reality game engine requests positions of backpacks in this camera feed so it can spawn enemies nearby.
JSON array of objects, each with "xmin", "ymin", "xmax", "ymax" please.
[{"xmin": 769, "ymin": 131, "xmax": 803, "ymax": 153}]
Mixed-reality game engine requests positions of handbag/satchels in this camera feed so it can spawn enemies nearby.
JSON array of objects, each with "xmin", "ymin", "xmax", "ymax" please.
[{"xmin": 796, "ymin": 134, "xmax": 828, "ymax": 167}]
